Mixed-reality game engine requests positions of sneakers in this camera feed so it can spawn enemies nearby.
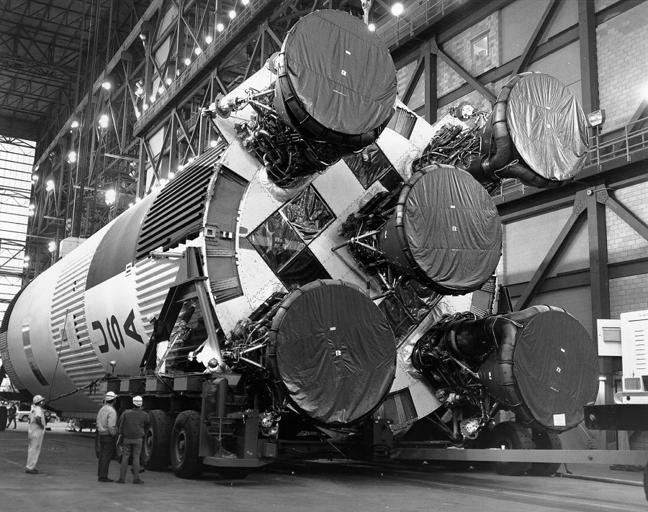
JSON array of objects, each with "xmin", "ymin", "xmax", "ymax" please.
[
  {"xmin": 98, "ymin": 477, "xmax": 113, "ymax": 482},
  {"xmin": 133, "ymin": 479, "xmax": 144, "ymax": 484},
  {"xmin": 116, "ymin": 480, "xmax": 124, "ymax": 483},
  {"xmin": 24, "ymin": 467, "xmax": 38, "ymax": 474}
]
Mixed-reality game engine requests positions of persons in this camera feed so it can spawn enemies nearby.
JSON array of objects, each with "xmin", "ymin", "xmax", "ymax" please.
[
  {"xmin": 116, "ymin": 396, "xmax": 150, "ymax": 483},
  {"xmin": 96, "ymin": 392, "xmax": 118, "ymax": 482},
  {"xmin": 6, "ymin": 402, "xmax": 16, "ymax": 429},
  {"xmin": 24, "ymin": 395, "xmax": 46, "ymax": 474}
]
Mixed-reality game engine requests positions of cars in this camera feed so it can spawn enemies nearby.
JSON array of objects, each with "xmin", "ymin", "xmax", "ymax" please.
[{"xmin": 18, "ymin": 410, "xmax": 58, "ymax": 423}]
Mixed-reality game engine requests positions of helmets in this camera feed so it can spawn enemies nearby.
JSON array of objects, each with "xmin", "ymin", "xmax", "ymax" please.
[
  {"xmin": 132, "ymin": 396, "xmax": 143, "ymax": 407},
  {"xmin": 33, "ymin": 395, "xmax": 45, "ymax": 403},
  {"xmin": 105, "ymin": 391, "xmax": 118, "ymax": 401}
]
